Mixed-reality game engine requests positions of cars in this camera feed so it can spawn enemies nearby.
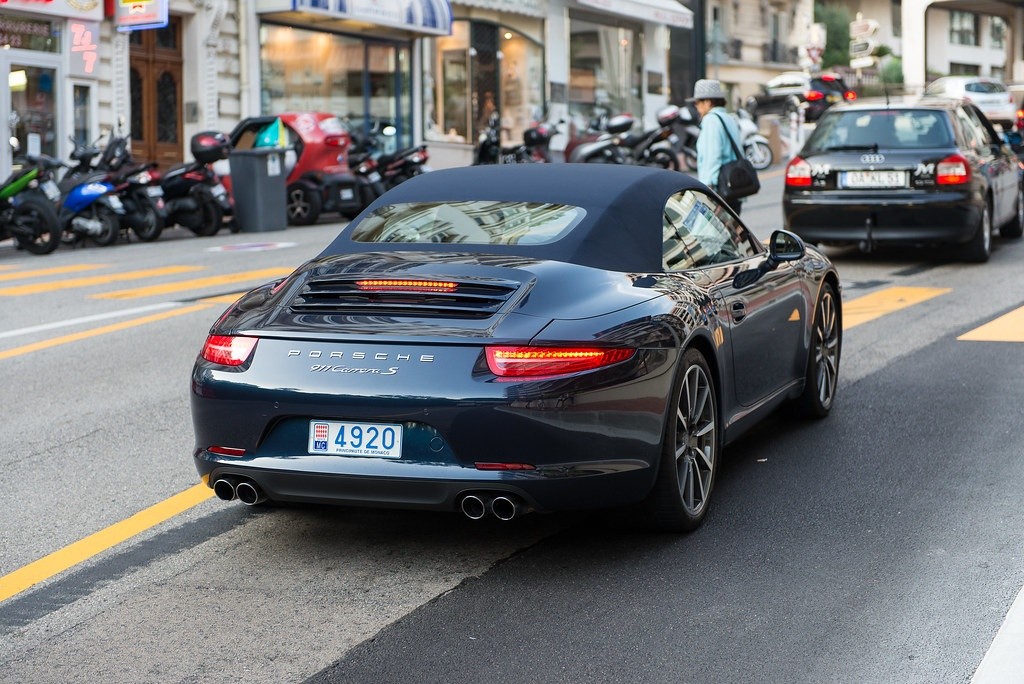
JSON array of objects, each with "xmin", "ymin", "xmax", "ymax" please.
[
  {"xmin": 744, "ymin": 71, "xmax": 858, "ymax": 123},
  {"xmin": 784, "ymin": 95, "xmax": 1024, "ymax": 263},
  {"xmin": 924, "ymin": 76, "xmax": 1016, "ymax": 131}
]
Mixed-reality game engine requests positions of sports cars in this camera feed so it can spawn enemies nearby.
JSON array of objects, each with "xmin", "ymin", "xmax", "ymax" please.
[{"xmin": 190, "ymin": 163, "xmax": 843, "ymax": 537}]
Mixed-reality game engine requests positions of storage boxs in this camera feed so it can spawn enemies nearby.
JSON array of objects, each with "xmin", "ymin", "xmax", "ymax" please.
[
  {"xmin": 191, "ymin": 132, "xmax": 232, "ymax": 163},
  {"xmin": 523, "ymin": 127, "xmax": 548, "ymax": 145},
  {"xmin": 606, "ymin": 113, "xmax": 634, "ymax": 134},
  {"xmin": 657, "ymin": 105, "xmax": 680, "ymax": 127}
]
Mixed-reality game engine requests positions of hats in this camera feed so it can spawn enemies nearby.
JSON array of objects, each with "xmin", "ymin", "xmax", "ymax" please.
[{"xmin": 684, "ymin": 80, "xmax": 730, "ymax": 102}]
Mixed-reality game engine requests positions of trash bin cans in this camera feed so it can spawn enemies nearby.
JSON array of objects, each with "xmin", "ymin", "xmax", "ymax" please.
[{"xmin": 222, "ymin": 116, "xmax": 294, "ymax": 232}]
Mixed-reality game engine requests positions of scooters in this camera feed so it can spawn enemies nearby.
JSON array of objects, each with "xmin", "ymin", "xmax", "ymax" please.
[
  {"xmin": 467, "ymin": 97, "xmax": 773, "ymax": 172},
  {"xmin": 0, "ymin": 107, "xmax": 429, "ymax": 255}
]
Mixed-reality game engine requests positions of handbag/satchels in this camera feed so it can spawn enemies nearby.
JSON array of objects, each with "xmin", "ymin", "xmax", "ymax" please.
[{"xmin": 716, "ymin": 157, "xmax": 761, "ymax": 200}]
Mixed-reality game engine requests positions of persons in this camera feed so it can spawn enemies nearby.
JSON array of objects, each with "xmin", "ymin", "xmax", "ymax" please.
[
  {"xmin": 425, "ymin": 119, "xmax": 466, "ymax": 143},
  {"xmin": 735, "ymin": 96, "xmax": 744, "ymax": 118},
  {"xmin": 684, "ymin": 79, "xmax": 745, "ymax": 217}
]
